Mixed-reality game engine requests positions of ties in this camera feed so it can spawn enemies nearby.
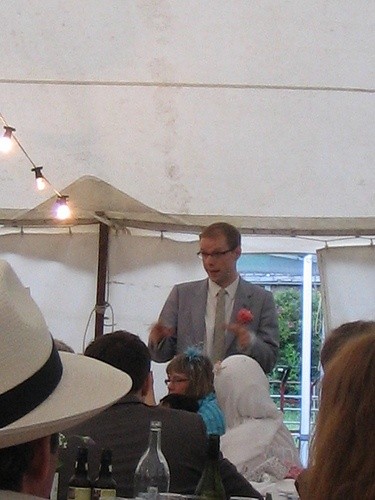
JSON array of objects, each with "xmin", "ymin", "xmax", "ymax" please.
[{"xmin": 212, "ymin": 289, "xmax": 228, "ymax": 366}]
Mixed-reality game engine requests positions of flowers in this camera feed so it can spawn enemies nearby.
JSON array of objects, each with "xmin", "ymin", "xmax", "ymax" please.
[
  {"xmin": 236, "ymin": 308, "xmax": 254, "ymax": 326},
  {"xmin": 184, "ymin": 340, "xmax": 204, "ymax": 363}
]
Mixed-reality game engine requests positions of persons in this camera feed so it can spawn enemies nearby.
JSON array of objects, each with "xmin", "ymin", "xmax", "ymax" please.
[
  {"xmin": 156, "ymin": 391, "xmax": 265, "ymax": 500},
  {"xmin": 213, "ymin": 354, "xmax": 301, "ymax": 480},
  {"xmin": 54, "ymin": 330, "xmax": 225, "ymax": 500},
  {"xmin": 293, "ymin": 320, "xmax": 375, "ymax": 500},
  {"xmin": 146, "ymin": 221, "xmax": 280, "ymax": 374},
  {"xmin": 0, "ymin": 259, "xmax": 133, "ymax": 500},
  {"xmin": 165, "ymin": 349, "xmax": 227, "ymax": 438}
]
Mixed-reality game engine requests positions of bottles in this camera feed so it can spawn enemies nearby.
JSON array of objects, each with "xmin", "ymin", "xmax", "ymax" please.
[
  {"xmin": 194, "ymin": 434, "xmax": 227, "ymax": 500},
  {"xmin": 64, "ymin": 447, "xmax": 92, "ymax": 500},
  {"xmin": 90, "ymin": 447, "xmax": 119, "ymax": 500},
  {"xmin": 133, "ymin": 420, "xmax": 170, "ymax": 500}
]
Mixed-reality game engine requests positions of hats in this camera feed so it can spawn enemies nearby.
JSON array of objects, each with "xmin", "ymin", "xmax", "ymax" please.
[{"xmin": 0, "ymin": 258, "xmax": 132, "ymax": 447}]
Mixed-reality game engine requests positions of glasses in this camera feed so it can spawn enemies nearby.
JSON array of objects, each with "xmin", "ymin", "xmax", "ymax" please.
[
  {"xmin": 164, "ymin": 379, "xmax": 188, "ymax": 387},
  {"xmin": 196, "ymin": 248, "xmax": 234, "ymax": 259}
]
